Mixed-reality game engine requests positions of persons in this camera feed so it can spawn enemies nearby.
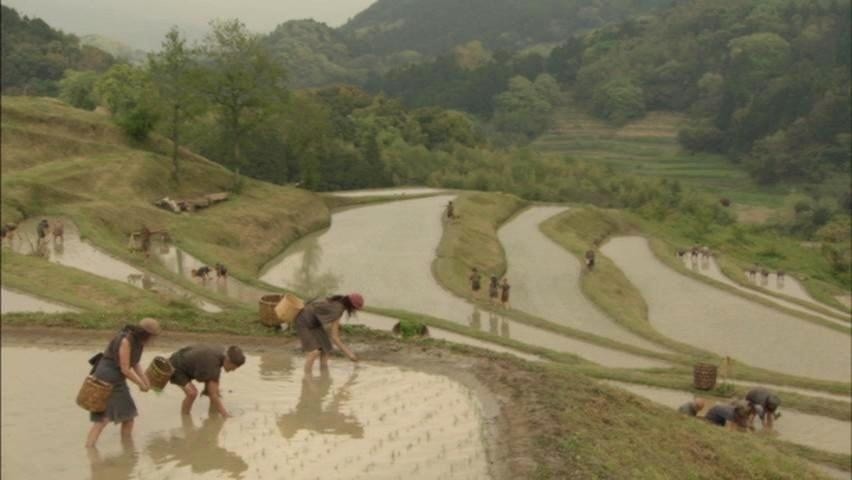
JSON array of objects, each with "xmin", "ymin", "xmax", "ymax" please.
[
  {"xmin": 166, "ymin": 346, "xmax": 245, "ymax": 417},
  {"xmin": 469, "ymin": 268, "xmax": 513, "ymax": 311},
  {"xmin": 82, "ymin": 317, "xmax": 162, "ymax": 448},
  {"xmin": 296, "ymin": 292, "xmax": 363, "ymax": 372},
  {"xmin": 442, "ymin": 201, "xmax": 456, "ymax": 225},
  {"xmin": 133, "ymin": 222, "xmax": 152, "ymax": 259},
  {"xmin": 679, "ymin": 386, "xmax": 781, "ymax": 433},
  {"xmin": 0, "ymin": 218, "xmax": 65, "ymax": 246},
  {"xmin": 584, "ymin": 247, "xmax": 595, "ymax": 272},
  {"xmin": 196, "ymin": 261, "xmax": 229, "ymax": 281}
]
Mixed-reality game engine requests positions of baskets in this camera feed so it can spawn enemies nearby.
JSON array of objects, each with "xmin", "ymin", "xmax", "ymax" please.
[
  {"xmin": 275, "ymin": 292, "xmax": 304, "ymax": 323},
  {"xmin": 76, "ymin": 376, "xmax": 113, "ymax": 413},
  {"xmin": 694, "ymin": 362, "xmax": 716, "ymax": 390},
  {"xmin": 144, "ymin": 356, "xmax": 172, "ymax": 391},
  {"xmin": 260, "ymin": 294, "xmax": 284, "ymax": 326}
]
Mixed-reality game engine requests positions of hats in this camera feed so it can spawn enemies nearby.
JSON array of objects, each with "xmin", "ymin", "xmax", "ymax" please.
[
  {"xmin": 228, "ymin": 345, "xmax": 244, "ymax": 366},
  {"xmin": 140, "ymin": 317, "xmax": 160, "ymax": 335},
  {"xmin": 349, "ymin": 293, "xmax": 363, "ymax": 309}
]
